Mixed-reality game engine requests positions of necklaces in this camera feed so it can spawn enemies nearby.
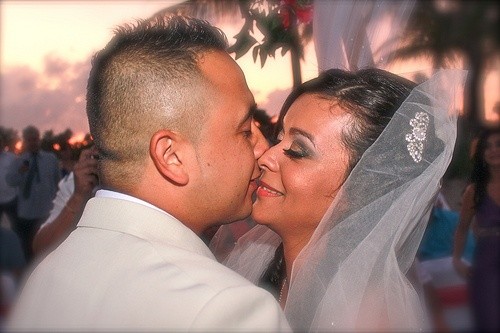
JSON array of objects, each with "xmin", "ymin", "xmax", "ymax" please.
[{"xmin": 279, "ymin": 279, "xmax": 287, "ymax": 303}]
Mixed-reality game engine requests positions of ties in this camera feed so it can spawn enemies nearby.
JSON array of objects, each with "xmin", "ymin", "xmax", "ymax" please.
[{"xmin": 22, "ymin": 153, "xmax": 40, "ymax": 200}]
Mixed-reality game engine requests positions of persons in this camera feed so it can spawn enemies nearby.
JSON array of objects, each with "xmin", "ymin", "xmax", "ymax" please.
[
  {"xmin": 453, "ymin": 127, "xmax": 500, "ymax": 333},
  {"xmin": 251, "ymin": 67, "xmax": 449, "ymax": 333},
  {"xmin": 0, "ymin": 123, "xmax": 101, "ymax": 271},
  {"xmin": 5, "ymin": 16, "xmax": 296, "ymax": 333}
]
